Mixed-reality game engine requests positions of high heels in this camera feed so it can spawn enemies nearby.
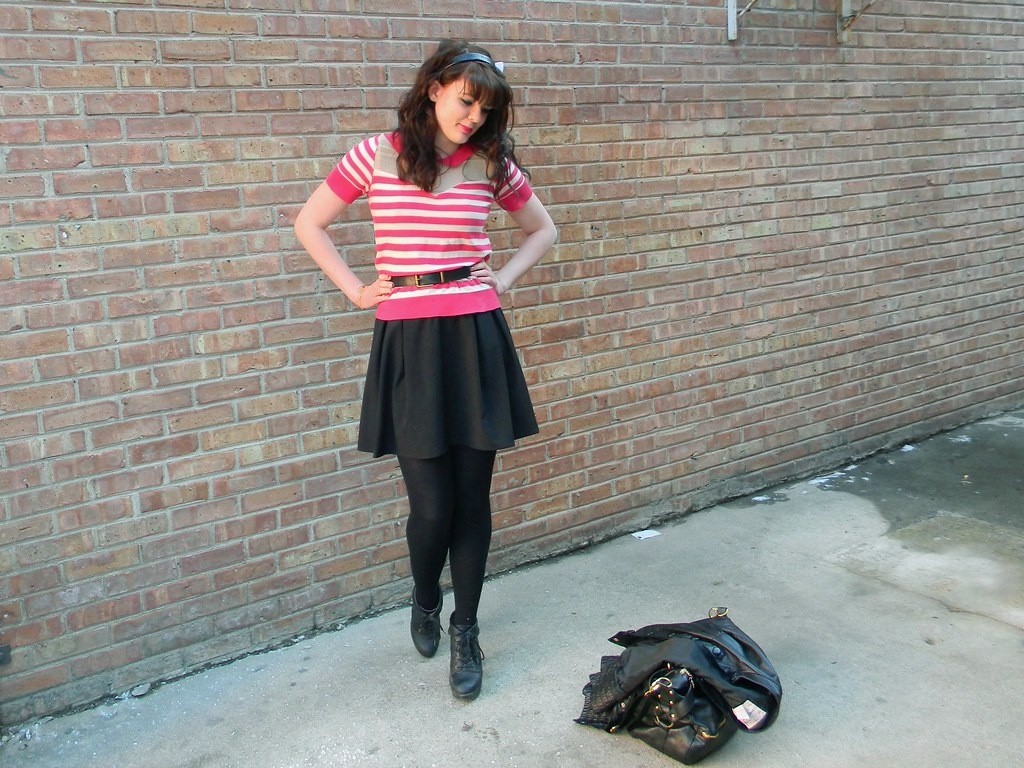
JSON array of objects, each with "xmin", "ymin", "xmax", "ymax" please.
[
  {"xmin": 411, "ymin": 585, "xmax": 445, "ymax": 657},
  {"xmin": 448, "ymin": 609, "xmax": 485, "ymax": 700}
]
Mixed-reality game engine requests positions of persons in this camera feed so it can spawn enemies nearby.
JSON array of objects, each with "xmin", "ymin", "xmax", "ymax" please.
[{"xmin": 294, "ymin": 38, "xmax": 558, "ymax": 701}]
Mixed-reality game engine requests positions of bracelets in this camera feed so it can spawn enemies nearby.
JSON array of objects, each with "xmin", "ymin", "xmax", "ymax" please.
[{"xmin": 359, "ymin": 288, "xmax": 364, "ymax": 307}]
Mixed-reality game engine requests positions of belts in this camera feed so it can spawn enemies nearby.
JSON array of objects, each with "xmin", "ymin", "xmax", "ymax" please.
[{"xmin": 389, "ymin": 268, "xmax": 472, "ymax": 287}]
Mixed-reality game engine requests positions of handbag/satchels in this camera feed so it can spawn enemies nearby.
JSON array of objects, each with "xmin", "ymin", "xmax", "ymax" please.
[{"xmin": 626, "ymin": 608, "xmax": 740, "ymax": 765}]
